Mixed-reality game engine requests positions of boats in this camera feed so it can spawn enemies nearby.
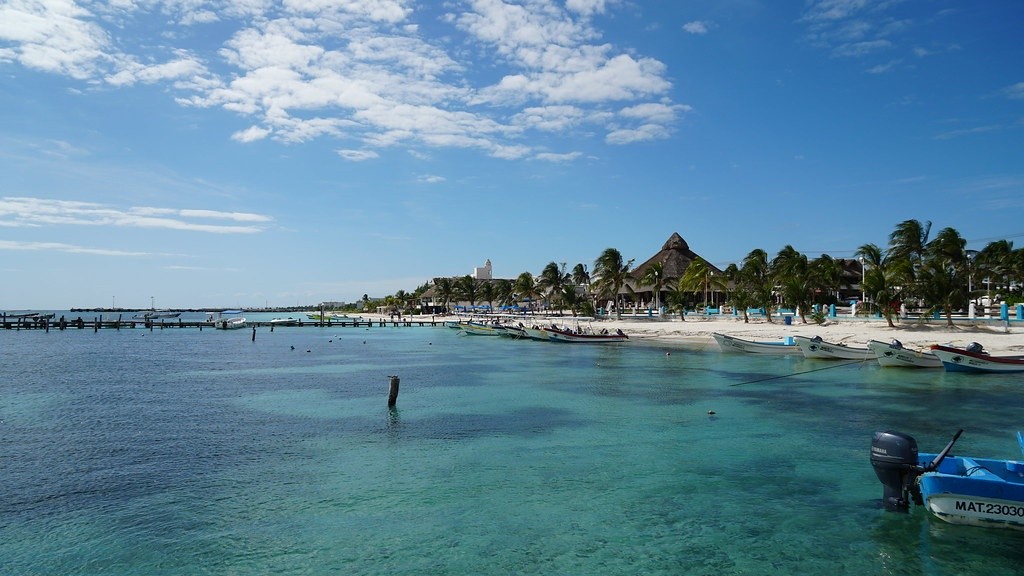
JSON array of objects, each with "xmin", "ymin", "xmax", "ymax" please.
[
  {"xmin": 0, "ymin": 312, "xmax": 40, "ymax": 318},
  {"xmin": 710, "ymin": 332, "xmax": 805, "ymax": 356},
  {"xmin": 159, "ymin": 312, "xmax": 182, "ymax": 318},
  {"xmin": 271, "ymin": 317, "xmax": 297, "ymax": 323},
  {"xmin": 132, "ymin": 313, "xmax": 159, "ymax": 319},
  {"xmin": 25, "ymin": 313, "xmax": 56, "ymax": 319},
  {"xmin": 540, "ymin": 325, "xmax": 629, "ymax": 344},
  {"xmin": 793, "ymin": 335, "xmax": 878, "ymax": 362},
  {"xmin": 868, "ymin": 430, "xmax": 1024, "ymax": 531},
  {"xmin": 445, "ymin": 321, "xmax": 553, "ymax": 341},
  {"xmin": 216, "ymin": 317, "xmax": 247, "ymax": 329},
  {"xmin": 864, "ymin": 338, "xmax": 943, "ymax": 368},
  {"xmin": 929, "ymin": 342, "xmax": 1024, "ymax": 374}
]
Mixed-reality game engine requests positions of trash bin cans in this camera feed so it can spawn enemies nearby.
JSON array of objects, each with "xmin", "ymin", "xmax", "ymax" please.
[{"xmin": 785, "ymin": 315, "xmax": 791, "ymax": 325}]
